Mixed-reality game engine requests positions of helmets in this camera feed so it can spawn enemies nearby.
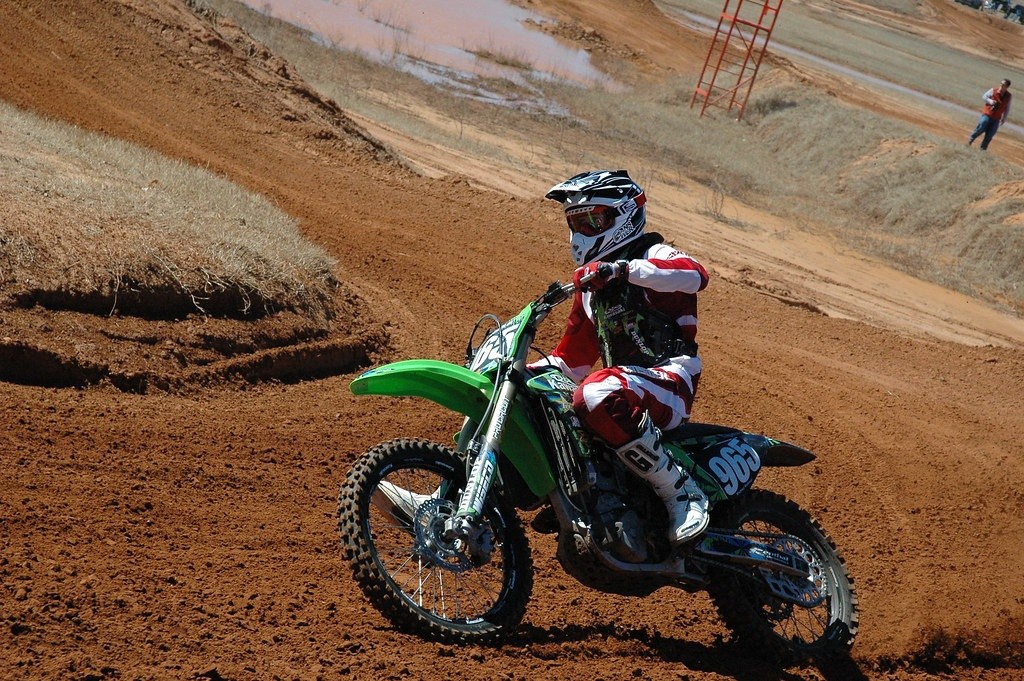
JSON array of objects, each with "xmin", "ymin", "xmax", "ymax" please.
[{"xmin": 545, "ymin": 170, "xmax": 647, "ymax": 266}]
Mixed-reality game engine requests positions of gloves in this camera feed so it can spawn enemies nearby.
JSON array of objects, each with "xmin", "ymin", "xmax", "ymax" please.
[{"xmin": 573, "ymin": 261, "xmax": 621, "ymax": 292}]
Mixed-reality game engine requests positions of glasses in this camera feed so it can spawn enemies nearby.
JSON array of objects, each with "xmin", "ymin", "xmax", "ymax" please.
[{"xmin": 568, "ymin": 213, "xmax": 613, "ymax": 235}]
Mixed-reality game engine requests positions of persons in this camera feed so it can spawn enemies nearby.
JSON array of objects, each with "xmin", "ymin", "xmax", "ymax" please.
[
  {"xmin": 526, "ymin": 167, "xmax": 708, "ymax": 544},
  {"xmin": 967, "ymin": 78, "xmax": 1012, "ymax": 150}
]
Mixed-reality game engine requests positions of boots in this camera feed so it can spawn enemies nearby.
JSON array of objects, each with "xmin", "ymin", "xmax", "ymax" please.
[
  {"xmin": 371, "ymin": 480, "xmax": 442, "ymax": 538},
  {"xmin": 615, "ymin": 409, "xmax": 713, "ymax": 544}
]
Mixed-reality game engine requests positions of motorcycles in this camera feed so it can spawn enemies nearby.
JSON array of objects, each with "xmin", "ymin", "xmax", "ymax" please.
[{"xmin": 334, "ymin": 263, "xmax": 861, "ymax": 670}]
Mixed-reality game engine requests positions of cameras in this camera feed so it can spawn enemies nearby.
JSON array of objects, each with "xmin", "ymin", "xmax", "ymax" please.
[{"xmin": 991, "ymin": 102, "xmax": 1001, "ymax": 112}]
